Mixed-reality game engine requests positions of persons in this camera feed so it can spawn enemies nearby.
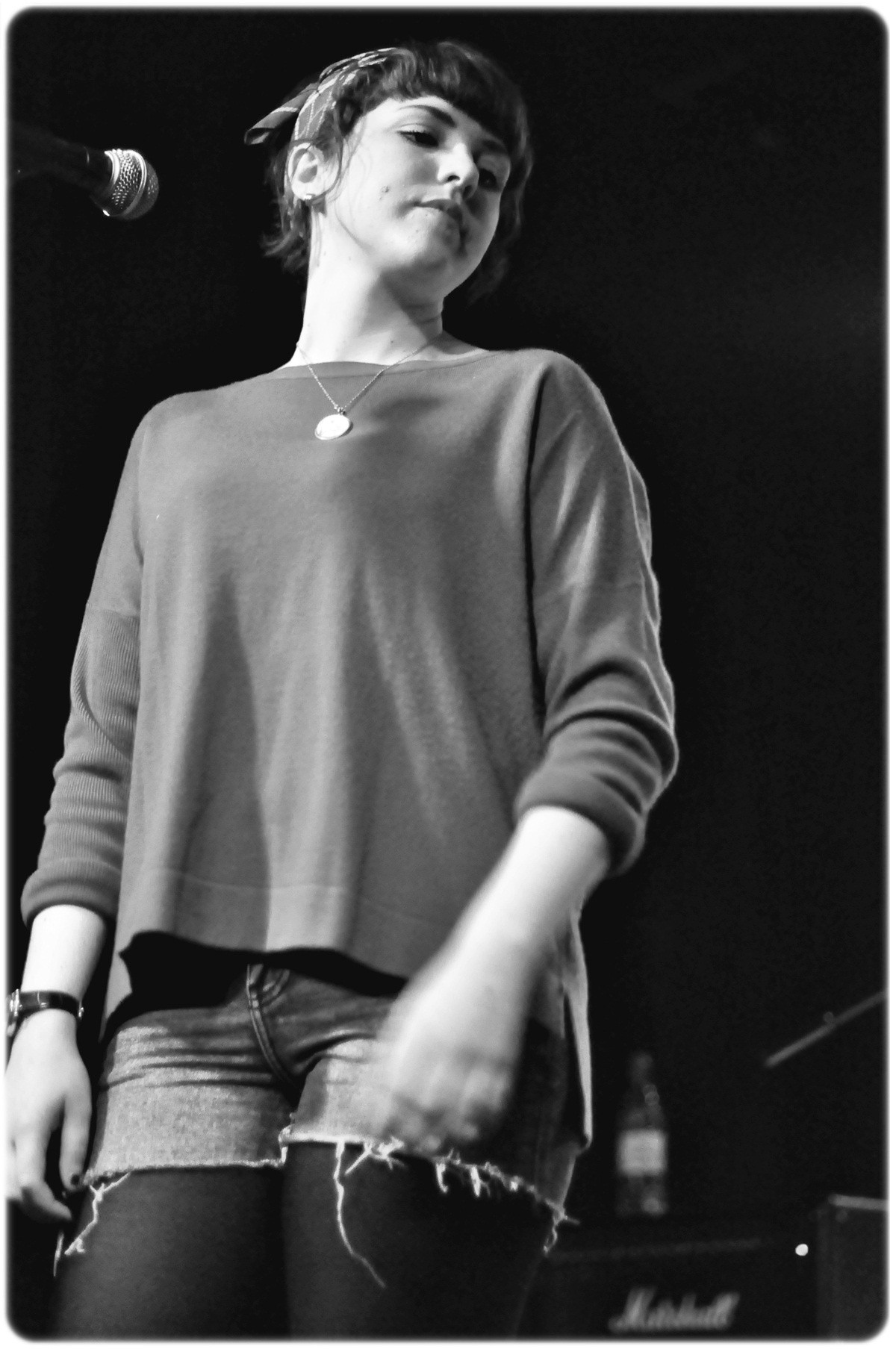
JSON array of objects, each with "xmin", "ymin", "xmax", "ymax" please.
[{"xmin": 6, "ymin": 33, "xmax": 684, "ymax": 1343}]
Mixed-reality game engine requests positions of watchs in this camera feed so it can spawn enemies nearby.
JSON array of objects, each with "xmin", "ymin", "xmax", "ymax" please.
[{"xmin": 7, "ymin": 988, "xmax": 83, "ymax": 1038}]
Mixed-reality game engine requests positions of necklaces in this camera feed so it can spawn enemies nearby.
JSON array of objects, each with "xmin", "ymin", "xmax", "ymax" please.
[{"xmin": 294, "ymin": 336, "xmax": 436, "ymax": 441}]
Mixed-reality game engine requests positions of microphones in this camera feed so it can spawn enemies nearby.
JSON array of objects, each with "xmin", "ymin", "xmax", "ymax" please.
[{"xmin": 9, "ymin": 120, "xmax": 159, "ymax": 220}]
